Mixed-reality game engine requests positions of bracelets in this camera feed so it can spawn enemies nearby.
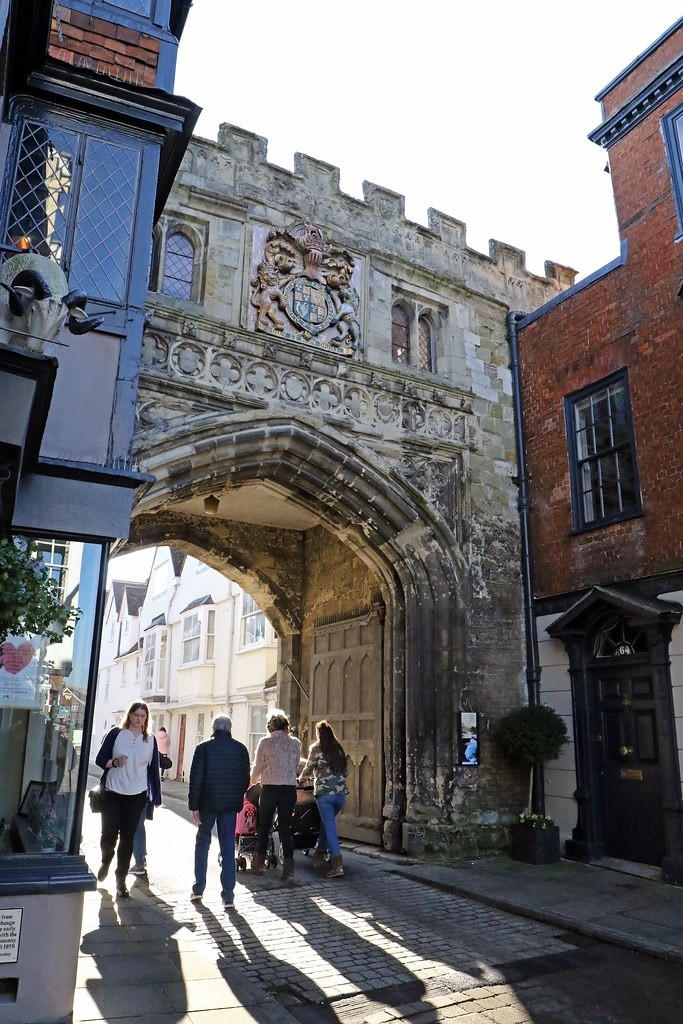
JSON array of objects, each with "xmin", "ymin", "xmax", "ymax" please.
[{"xmin": 112, "ymin": 759, "xmax": 120, "ymax": 768}]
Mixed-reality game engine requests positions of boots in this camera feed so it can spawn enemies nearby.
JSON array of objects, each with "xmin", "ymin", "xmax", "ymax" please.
[
  {"xmin": 312, "ymin": 847, "xmax": 325, "ymax": 868},
  {"xmin": 327, "ymin": 855, "xmax": 344, "ymax": 877},
  {"xmin": 280, "ymin": 858, "xmax": 295, "ymax": 883},
  {"xmin": 251, "ymin": 852, "xmax": 267, "ymax": 875}
]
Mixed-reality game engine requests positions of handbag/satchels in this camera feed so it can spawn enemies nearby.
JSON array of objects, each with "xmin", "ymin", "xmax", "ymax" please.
[{"xmin": 88, "ymin": 785, "xmax": 101, "ymax": 813}]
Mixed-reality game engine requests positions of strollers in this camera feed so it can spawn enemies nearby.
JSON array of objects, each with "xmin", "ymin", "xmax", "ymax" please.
[
  {"xmin": 272, "ymin": 776, "xmax": 331, "ymax": 867},
  {"xmin": 218, "ymin": 784, "xmax": 277, "ymax": 872}
]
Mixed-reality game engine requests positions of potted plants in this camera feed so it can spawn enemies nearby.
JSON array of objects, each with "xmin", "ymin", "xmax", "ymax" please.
[{"xmin": 497, "ymin": 705, "xmax": 571, "ymax": 867}]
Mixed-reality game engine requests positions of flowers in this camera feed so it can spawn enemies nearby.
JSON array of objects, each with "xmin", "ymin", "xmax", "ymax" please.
[
  {"xmin": 517, "ymin": 813, "xmax": 553, "ymax": 830},
  {"xmin": 0, "ymin": 536, "xmax": 83, "ymax": 641},
  {"xmin": 29, "ymin": 806, "xmax": 68, "ymax": 845}
]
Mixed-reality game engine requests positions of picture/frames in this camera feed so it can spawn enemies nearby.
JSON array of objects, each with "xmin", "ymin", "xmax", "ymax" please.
[
  {"xmin": 457, "ymin": 710, "xmax": 481, "ymax": 766},
  {"xmin": 17, "ymin": 779, "xmax": 48, "ymax": 819}
]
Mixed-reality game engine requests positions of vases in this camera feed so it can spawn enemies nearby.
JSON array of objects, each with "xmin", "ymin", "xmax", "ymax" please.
[{"xmin": 40, "ymin": 845, "xmax": 56, "ymax": 853}]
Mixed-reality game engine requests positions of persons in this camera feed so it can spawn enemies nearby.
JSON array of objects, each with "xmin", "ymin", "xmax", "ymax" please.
[
  {"xmin": 95, "ymin": 702, "xmax": 162, "ymax": 898},
  {"xmin": 249, "ymin": 714, "xmax": 301, "ymax": 883},
  {"xmin": 156, "ymin": 727, "xmax": 170, "ymax": 781},
  {"xmin": 128, "ymin": 752, "xmax": 173, "ymax": 875},
  {"xmin": 297, "ymin": 723, "xmax": 350, "ymax": 878},
  {"xmin": 189, "ymin": 715, "xmax": 251, "ymax": 907}
]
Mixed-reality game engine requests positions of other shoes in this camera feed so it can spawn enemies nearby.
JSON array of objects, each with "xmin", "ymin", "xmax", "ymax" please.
[
  {"xmin": 161, "ymin": 776, "xmax": 164, "ymax": 781},
  {"xmin": 116, "ymin": 886, "xmax": 129, "ymax": 897},
  {"xmin": 143, "ymin": 857, "xmax": 147, "ymax": 867},
  {"xmin": 128, "ymin": 864, "xmax": 145, "ymax": 874},
  {"xmin": 222, "ymin": 900, "xmax": 234, "ymax": 908},
  {"xmin": 189, "ymin": 892, "xmax": 202, "ymax": 900},
  {"xmin": 98, "ymin": 852, "xmax": 114, "ymax": 882}
]
